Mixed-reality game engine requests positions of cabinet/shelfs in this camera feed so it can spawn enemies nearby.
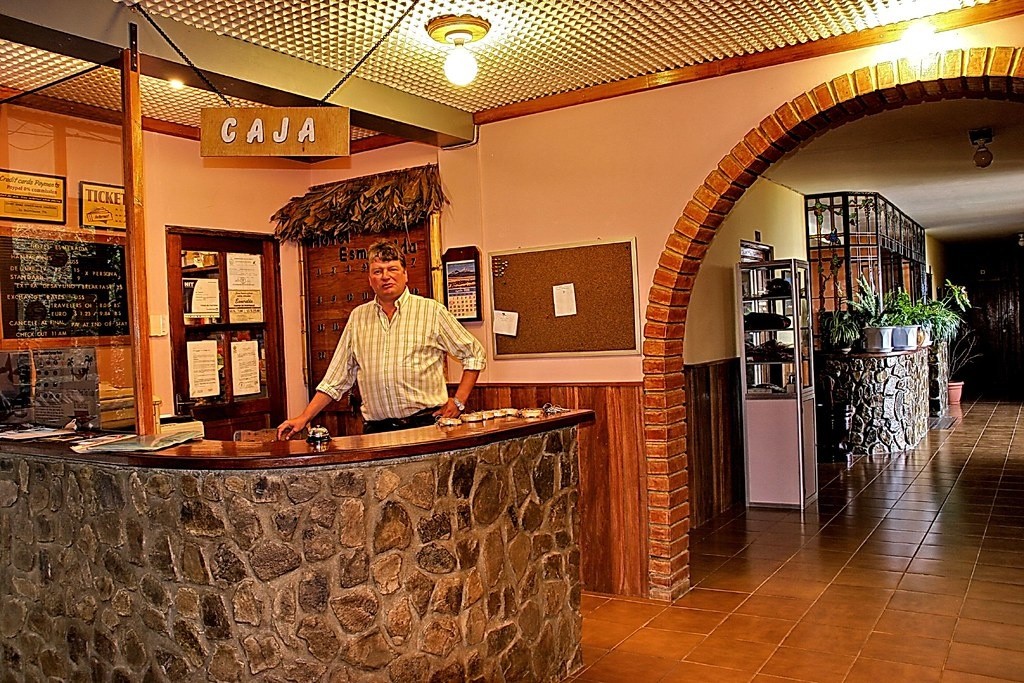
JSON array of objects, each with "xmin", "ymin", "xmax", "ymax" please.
[{"xmin": 733, "ymin": 259, "xmax": 818, "ymax": 512}]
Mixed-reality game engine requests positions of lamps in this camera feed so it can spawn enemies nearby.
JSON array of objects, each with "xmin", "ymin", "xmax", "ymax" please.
[
  {"xmin": 421, "ymin": 14, "xmax": 493, "ymax": 91},
  {"xmin": 968, "ymin": 128, "xmax": 997, "ymax": 171}
]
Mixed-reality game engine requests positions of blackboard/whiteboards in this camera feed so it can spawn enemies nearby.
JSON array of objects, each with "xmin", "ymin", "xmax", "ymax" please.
[{"xmin": 0, "ymin": 218, "xmax": 132, "ymax": 350}]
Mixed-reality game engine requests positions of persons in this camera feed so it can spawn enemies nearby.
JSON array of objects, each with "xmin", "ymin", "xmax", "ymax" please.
[{"xmin": 275, "ymin": 241, "xmax": 486, "ymax": 441}]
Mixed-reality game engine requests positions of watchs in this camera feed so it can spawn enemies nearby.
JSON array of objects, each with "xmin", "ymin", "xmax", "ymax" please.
[{"xmin": 452, "ymin": 397, "xmax": 465, "ymax": 411}]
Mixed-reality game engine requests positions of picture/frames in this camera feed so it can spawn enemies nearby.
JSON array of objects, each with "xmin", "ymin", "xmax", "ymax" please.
[
  {"xmin": 78, "ymin": 180, "xmax": 126, "ymax": 232},
  {"xmin": 0, "ymin": 168, "xmax": 67, "ymax": 226}
]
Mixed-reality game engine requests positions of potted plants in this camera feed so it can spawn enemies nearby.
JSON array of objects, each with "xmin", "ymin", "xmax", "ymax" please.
[
  {"xmin": 842, "ymin": 271, "xmax": 902, "ymax": 354},
  {"xmin": 914, "ymin": 296, "xmax": 944, "ymax": 348},
  {"xmin": 948, "ymin": 330, "xmax": 983, "ymax": 405},
  {"xmin": 927, "ymin": 299, "xmax": 966, "ymax": 346},
  {"xmin": 824, "ymin": 309, "xmax": 861, "ymax": 354},
  {"xmin": 884, "ymin": 286, "xmax": 928, "ymax": 351}
]
